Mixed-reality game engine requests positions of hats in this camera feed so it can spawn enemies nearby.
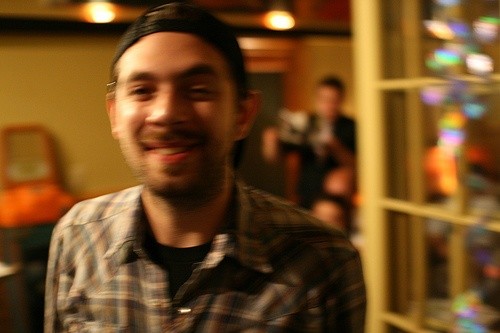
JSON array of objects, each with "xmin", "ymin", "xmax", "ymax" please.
[{"xmin": 109, "ymin": 1, "xmax": 249, "ymax": 99}]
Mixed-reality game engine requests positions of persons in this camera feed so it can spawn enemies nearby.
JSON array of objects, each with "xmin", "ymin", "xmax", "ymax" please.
[
  {"xmin": 263, "ymin": 74, "xmax": 359, "ymax": 234},
  {"xmin": 44, "ymin": 0, "xmax": 366, "ymax": 333}
]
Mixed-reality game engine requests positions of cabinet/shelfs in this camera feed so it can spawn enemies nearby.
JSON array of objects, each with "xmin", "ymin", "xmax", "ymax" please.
[{"xmin": 349, "ymin": 0, "xmax": 500, "ymax": 333}]
